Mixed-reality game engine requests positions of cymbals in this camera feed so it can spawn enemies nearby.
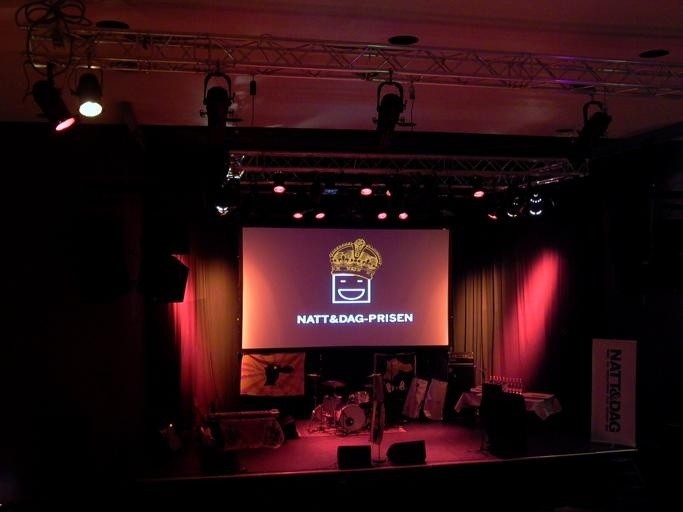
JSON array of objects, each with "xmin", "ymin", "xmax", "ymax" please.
[{"xmin": 322, "ymin": 381, "xmax": 344, "ymax": 387}]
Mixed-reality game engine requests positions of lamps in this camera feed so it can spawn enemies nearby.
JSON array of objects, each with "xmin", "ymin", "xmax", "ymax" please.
[
  {"xmin": 564, "ymin": 90, "xmax": 607, "ymax": 165},
  {"xmin": 76, "ymin": 71, "xmax": 104, "ymax": 119},
  {"xmin": 29, "ymin": 68, "xmax": 76, "ymax": 133},
  {"xmin": 375, "ymin": 73, "xmax": 404, "ymax": 137},
  {"xmin": 196, "ymin": 73, "xmax": 236, "ymax": 137}
]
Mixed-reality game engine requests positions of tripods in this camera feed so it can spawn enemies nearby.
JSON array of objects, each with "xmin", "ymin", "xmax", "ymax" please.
[{"xmin": 307, "ymin": 386, "xmax": 347, "ymax": 436}]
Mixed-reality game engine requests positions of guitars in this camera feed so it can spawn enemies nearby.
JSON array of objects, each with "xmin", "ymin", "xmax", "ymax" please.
[{"xmin": 373, "ymin": 374, "xmax": 384, "ymax": 444}]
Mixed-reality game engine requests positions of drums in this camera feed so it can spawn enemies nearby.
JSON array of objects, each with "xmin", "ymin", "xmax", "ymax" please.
[
  {"xmin": 349, "ymin": 392, "xmax": 354, "ymax": 402},
  {"xmin": 354, "ymin": 391, "xmax": 368, "ymax": 404},
  {"xmin": 337, "ymin": 404, "xmax": 366, "ymax": 432}
]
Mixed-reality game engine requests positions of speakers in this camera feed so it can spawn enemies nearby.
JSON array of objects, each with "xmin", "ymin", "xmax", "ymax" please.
[
  {"xmin": 387, "ymin": 441, "xmax": 426, "ymax": 465},
  {"xmin": 337, "ymin": 445, "xmax": 372, "ymax": 467}
]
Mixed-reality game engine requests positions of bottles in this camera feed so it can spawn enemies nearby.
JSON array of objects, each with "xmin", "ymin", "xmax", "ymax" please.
[{"xmin": 489, "ymin": 376, "xmax": 523, "ymax": 395}]
